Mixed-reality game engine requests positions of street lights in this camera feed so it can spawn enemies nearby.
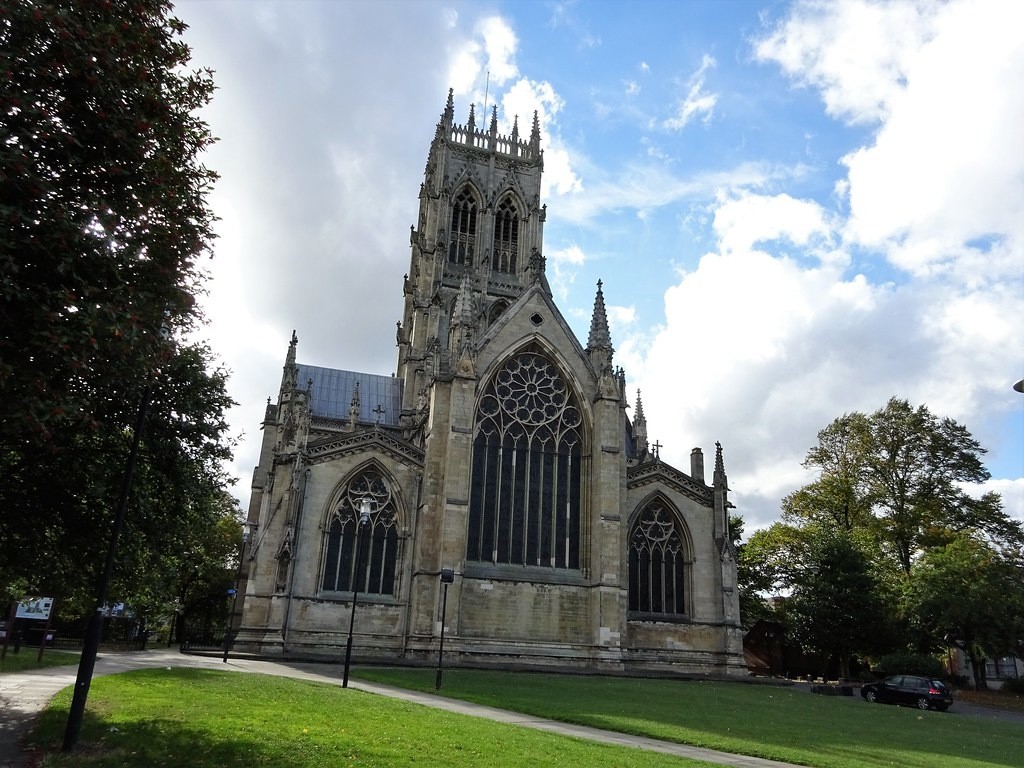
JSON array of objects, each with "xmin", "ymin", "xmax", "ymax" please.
[
  {"xmin": 342, "ymin": 492, "xmax": 374, "ymax": 687},
  {"xmin": 223, "ymin": 521, "xmax": 259, "ymax": 662}
]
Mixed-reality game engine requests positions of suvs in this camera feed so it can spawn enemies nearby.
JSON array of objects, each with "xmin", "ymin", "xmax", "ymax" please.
[{"xmin": 861, "ymin": 674, "xmax": 955, "ymax": 711}]
